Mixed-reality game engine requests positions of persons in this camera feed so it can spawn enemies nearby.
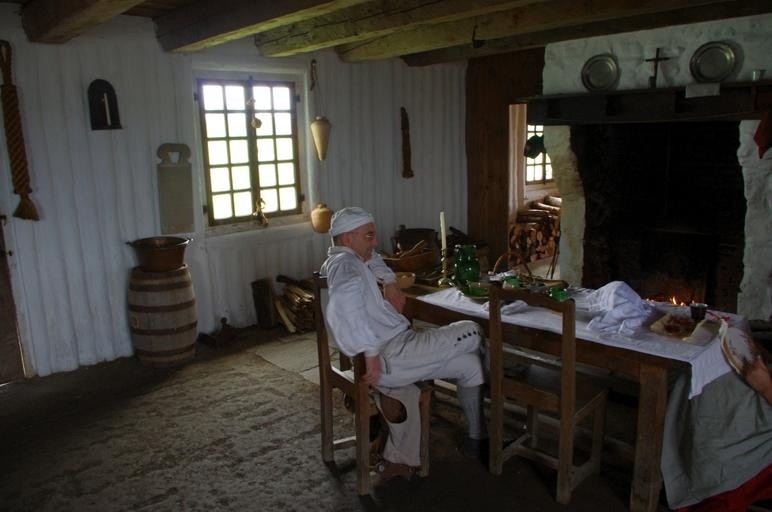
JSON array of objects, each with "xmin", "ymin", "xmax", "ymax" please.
[
  {"xmin": 320, "ymin": 204, "xmax": 489, "ymax": 447},
  {"xmin": 660, "ymin": 353, "xmax": 771, "ymax": 511}
]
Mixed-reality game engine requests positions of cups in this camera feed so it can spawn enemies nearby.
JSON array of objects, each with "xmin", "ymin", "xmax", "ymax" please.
[
  {"xmin": 530, "ymin": 281, "xmax": 569, "ymax": 300},
  {"xmin": 503, "ymin": 276, "xmax": 519, "ymax": 288},
  {"xmin": 691, "ymin": 302, "xmax": 708, "ymax": 324},
  {"xmin": 751, "ymin": 69, "xmax": 766, "ymax": 82}
]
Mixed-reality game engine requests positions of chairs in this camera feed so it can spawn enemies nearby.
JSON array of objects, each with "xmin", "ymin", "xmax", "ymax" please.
[
  {"xmin": 315, "ymin": 276, "xmax": 430, "ymax": 483},
  {"xmin": 485, "ymin": 278, "xmax": 617, "ymax": 503}
]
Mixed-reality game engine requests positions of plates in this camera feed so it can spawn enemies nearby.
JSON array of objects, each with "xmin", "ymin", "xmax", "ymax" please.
[
  {"xmin": 690, "ymin": 40, "xmax": 737, "ymax": 80},
  {"xmin": 579, "ymin": 52, "xmax": 619, "ymax": 90},
  {"xmin": 461, "ymin": 287, "xmax": 495, "ymax": 302}
]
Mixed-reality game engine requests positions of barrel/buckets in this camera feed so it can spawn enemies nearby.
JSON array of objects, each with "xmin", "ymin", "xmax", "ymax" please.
[{"xmin": 127, "ymin": 263, "xmax": 197, "ymax": 370}]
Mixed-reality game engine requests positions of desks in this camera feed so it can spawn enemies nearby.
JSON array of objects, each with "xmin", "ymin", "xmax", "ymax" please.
[{"xmin": 393, "ymin": 266, "xmax": 752, "ymax": 493}]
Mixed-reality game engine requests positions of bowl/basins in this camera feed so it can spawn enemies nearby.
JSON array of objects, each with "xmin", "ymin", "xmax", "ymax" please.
[{"xmin": 128, "ymin": 236, "xmax": 190, "ymax": 272}]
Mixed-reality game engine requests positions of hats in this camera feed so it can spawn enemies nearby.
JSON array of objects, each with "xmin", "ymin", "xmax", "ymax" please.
[{"xmin": 328, "ymin": 204, "xmax": 374, "ymax": 238}]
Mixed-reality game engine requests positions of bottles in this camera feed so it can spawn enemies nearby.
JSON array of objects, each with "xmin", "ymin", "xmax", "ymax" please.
[{"xmin": 451, "ymin": 244, "xmax": 482, "ymax": 291}]
